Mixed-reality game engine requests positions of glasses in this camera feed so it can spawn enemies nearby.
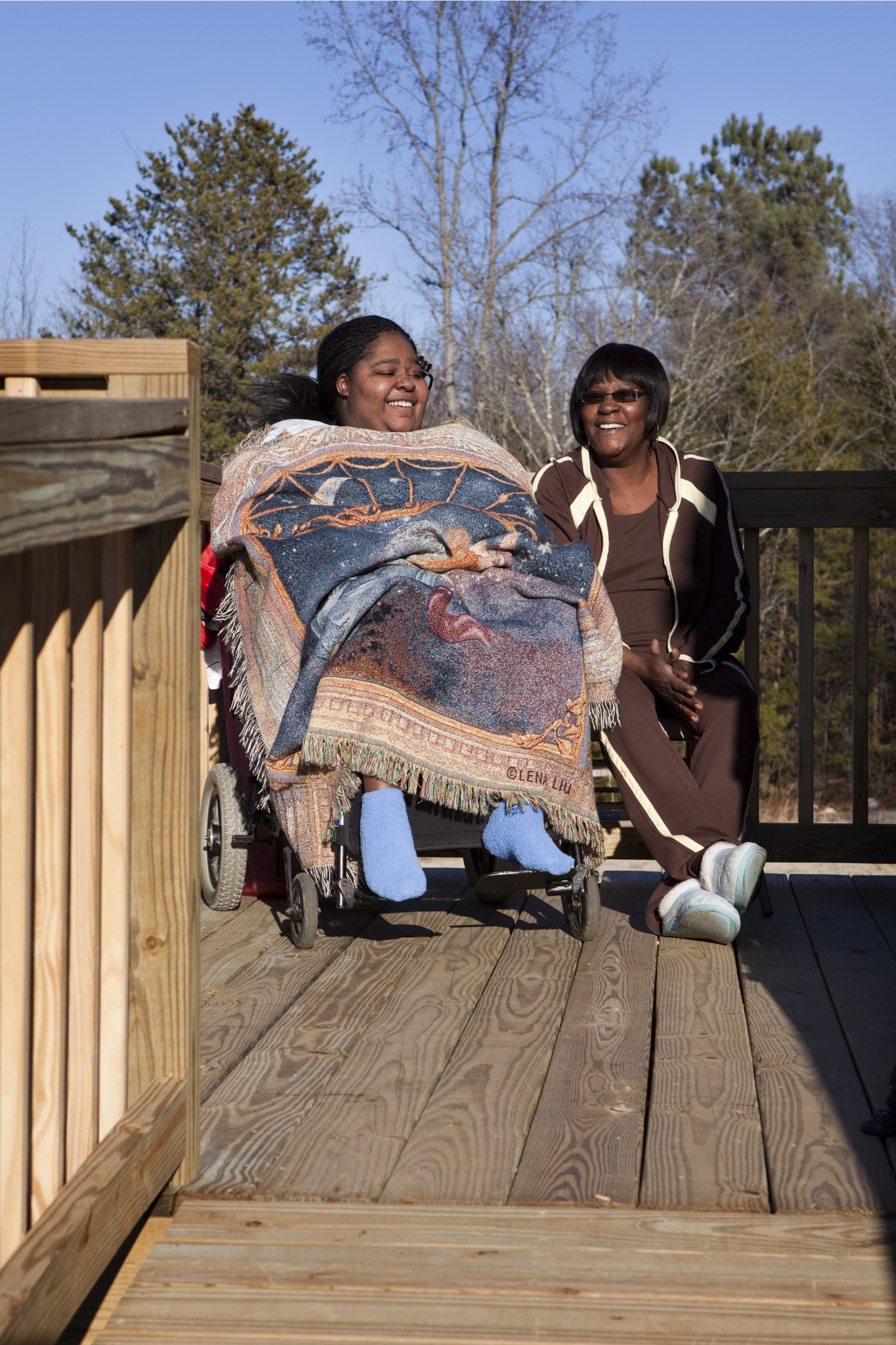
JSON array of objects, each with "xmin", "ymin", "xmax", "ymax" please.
[{"xmin": 580, "ymin": 389, "xmax": 645, "ymax": 405}]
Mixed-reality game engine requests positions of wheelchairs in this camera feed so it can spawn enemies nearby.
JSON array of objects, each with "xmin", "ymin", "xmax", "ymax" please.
[{"xmin": 195, "ymin": 624, "xmax": 603, "ymax": 950}]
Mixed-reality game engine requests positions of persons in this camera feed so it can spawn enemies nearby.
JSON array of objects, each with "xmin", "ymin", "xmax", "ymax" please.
[
  {"xmin": 258, "ymin": 316, "xmax": 576, "ymax": 901},
  {"xmin": 531, "ymin": 343, "xmax": 767, "ymax": 945}
]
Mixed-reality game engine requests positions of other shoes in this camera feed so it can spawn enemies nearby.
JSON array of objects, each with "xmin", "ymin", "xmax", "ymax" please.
[
  {"xmin": 699, "ymin": 840, "xmax": 767, "ymax": 920},
  {"xmin": 659, "ymin": 879, "xmax": 740, "ymax": 944}
]
